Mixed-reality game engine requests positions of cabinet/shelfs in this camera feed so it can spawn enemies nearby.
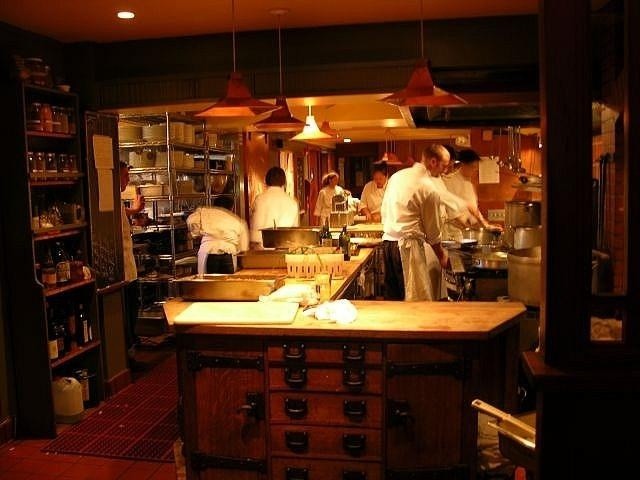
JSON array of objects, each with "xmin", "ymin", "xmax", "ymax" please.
[
  {"xmin": 208, "ymin": 148, "xmax": 236, "ymax": 217},
  {"xmin": 0, "ymin": 80, "xmax": 106, "ymax": 437},
  {"xmin": 118, "ymin": 111, "xmax": 207, "ymax": 348}
]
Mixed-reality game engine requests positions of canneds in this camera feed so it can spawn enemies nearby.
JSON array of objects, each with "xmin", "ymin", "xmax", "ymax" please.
[
  {"xmin": 40, "ymin": 101, "xmax": 53, "ymax": 132},
  {"xmin": 30, "ymin": 103, "xmax": 41, "ymax": 132},
  {"xmin": 52, "ymin": 106, "xmax": 77, "ymax": 133},
  {"xmin": 28, "ymin": 151, "xmax": 80, "ymax": 180}
]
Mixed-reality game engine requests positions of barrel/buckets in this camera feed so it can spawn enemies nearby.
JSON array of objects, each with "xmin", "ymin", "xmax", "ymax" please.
[{"xmin": 51, "ymin": 375, "xmax": 83, "ymax": 419}]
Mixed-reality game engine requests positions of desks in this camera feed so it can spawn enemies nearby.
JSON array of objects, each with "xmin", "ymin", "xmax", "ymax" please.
[{"xmin": 162, "ymin": 227, "xmax": 526, "ymax": 479}]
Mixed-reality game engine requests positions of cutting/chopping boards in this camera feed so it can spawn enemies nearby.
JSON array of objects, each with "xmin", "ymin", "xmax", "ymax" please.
[{"xmin": 173, "ymin": 302, "xmax": 299, "ymax": 323}]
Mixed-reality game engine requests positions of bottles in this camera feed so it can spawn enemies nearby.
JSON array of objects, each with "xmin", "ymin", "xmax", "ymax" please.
[
  {"xmin": 41, "ymin": 243, "xmax": 57, "ymax": 287},
  {"xmin": 56, "ymin": 239, "xmax": 71, "ymax": 286},
  {"xmin": 320, "ymin": 225, "xmax": 332, "ymax": 245},
  {"xmin": 340, "ymin": 224, "xmax": 349, "ymax": 261},
  {"xmin": 54, "ymin": 322, "xmax": 71, "ymax": 355},
  {"xmin": 45, "ymin": 303, "xmax": 59, "ymax": 362},
  {"xmin": 75, "ymin": 300, "xmax": 89, "ymax": 345}
]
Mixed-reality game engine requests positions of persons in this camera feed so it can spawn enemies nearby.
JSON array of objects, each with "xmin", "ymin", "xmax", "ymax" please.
[
  {"xmin": 431, "ymin": 143, "xmax": 506, "ymax": 243},
  {"xmin": 382, "ymin": 143, "xmax": 449, "ymax": 299},
  {"xmin": 185, "ymin": 196, "xmax": 251, "ymax": 276},
  {"xmin": 250, "ymin": 165, "xmax": 300, "ymax": 243},
  {"xmin": 314, "ymin": 172, "xmax": 345, "ymax": 225},
  {"xmin": 118, "ymin": 161, "xmax": 147, "ymax": 373},
  {"xmin": 442, "ymin": 148, "xmax": 483, "ymax": 224},
  {"xmin": 358, "ymin": 161, "xmax": 391, "ymax": 223}
]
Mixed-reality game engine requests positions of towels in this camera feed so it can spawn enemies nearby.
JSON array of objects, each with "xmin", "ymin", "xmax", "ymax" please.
[
  {"xmin": 304, "ymin": 299, "xmax": 358, "ymax": 325},
  {"xmin": 271, "ymin": 284, "xmax": 319, "ymax": 306}
]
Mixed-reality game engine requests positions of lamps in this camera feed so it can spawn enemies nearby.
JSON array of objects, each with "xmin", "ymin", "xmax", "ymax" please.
[
  {"xmin": 373, "ymin": 130, "xmax": 402, "ymax": 166},
  {"xmin": 308, "ymin": 120, "xmax": 343, "ymax": 142},
  {"xmin": 190, "ymin": 0, "xmax": 282, "ymax": 118},
  {"xmin": 376, "ymin": 0, "xmax": 468, "ymax": 107},
  {"xmin": 289, "ymin": 107, "xmax": 332, "ymax": 141},
  {"xmin": 251, "ymin": 10, "xmax": 311, "ymax": 133}
]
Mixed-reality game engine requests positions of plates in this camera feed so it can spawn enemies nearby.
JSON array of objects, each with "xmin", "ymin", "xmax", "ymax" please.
[
  {"xmin": 117, "ymin": 122, "xmax": 217, "ymax": 146},
  {"xmin": 128, "ymin": 150, "xmax": 204, "ymax": 168},
  {"xmin": 137, "ymin": 180, "xmax": 193, "ymax": 196}
]
